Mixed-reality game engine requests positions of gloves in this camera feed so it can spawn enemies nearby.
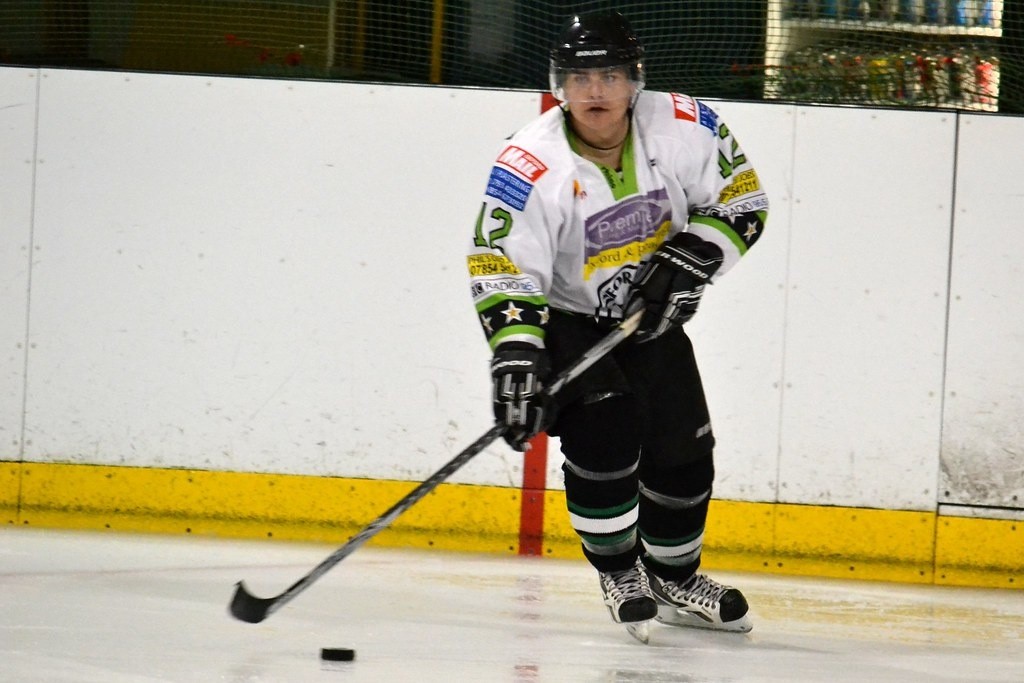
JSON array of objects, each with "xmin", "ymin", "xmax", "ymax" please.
[
  {"xmin": 621, "ymin": 229, "xmax": 724, "ymax": 343},
  {"xmin": 493, "ymin": 340, "xmax": 561, "ymax": 453}
]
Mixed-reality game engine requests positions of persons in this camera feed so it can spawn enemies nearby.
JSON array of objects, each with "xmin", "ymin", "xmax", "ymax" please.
[{"xmin": 467, "ymin": 10, "xmax": 769, "ymax": 645}]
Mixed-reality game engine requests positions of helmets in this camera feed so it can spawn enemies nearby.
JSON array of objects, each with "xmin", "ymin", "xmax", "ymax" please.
[{"xmin": 552, "ymin": 10, "xmax": 646, "ymax": 73}]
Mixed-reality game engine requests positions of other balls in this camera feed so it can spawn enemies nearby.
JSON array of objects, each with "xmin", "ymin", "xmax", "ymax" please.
[{"xmin": 319, "ymin": 647, "xmax": 357, "ymax": 663}]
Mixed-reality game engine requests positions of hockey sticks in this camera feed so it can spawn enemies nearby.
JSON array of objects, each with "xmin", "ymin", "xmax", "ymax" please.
[{"xmin": 220, "ymin": 304, "xmax": 649, "ymax": 627}]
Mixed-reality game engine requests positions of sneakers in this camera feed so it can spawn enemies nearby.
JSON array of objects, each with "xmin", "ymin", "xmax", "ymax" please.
[
  {"xmin": 640, "ymin": 557, "xmax": 752, "ymax": 634},
  {"xmin": 599, "ymin": 561, "xmax": 657, "ymax": 645}
]
